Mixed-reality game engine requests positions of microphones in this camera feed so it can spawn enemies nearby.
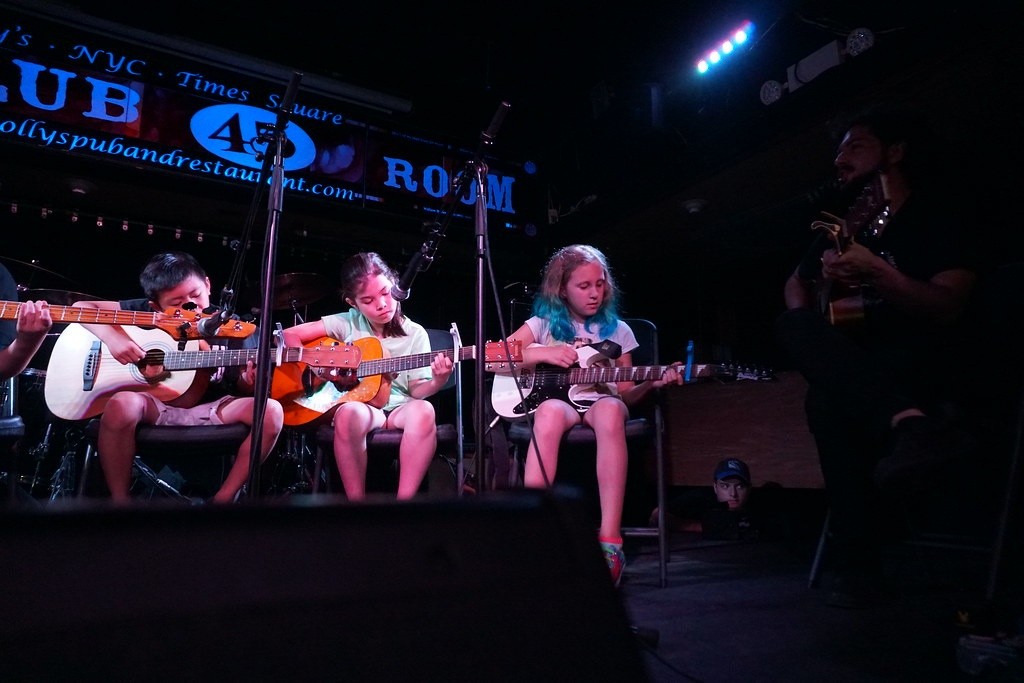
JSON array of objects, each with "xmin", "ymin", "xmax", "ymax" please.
[
  {"xmin": 391, "ymin": 240, "xmax": 432, "ymax": 301},
  {"xmin": 197, "ymin": 307, "xmax": 237, "ymax": 336}
]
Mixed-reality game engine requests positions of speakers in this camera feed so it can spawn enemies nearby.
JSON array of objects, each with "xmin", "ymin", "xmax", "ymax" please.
[{"xmin": 0, "ymin": 487, "xmax": 650, "ymax": 683}]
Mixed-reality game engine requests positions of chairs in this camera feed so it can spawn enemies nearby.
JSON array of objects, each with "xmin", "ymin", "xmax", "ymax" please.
[{"xmin": 66, "ymin": 299, "xmax": 667, "ymax": 590}]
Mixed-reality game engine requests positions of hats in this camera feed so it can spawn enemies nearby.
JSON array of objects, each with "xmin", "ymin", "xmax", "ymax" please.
[{"xmin": 714, "ymin": 458, "xmax": 751, "ymax": 486}]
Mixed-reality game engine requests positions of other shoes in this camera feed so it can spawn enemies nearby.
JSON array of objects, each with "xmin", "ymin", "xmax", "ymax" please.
[{"xmin": 599, "ymin": 535, "xmax": 626, "ymax": 587}]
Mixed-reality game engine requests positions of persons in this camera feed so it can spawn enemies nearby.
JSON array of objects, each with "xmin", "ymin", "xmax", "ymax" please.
[
  {"xmin": 784, "ymin": 119, "xmax": 1006, "ymax": 633},
  {"xmin": 273, "ymin": 252, "xmax": 454, "ymax": 500},
  {"xmin": 485, "ymin": 245, "xmax": 684, "ymax": 587},
  {"xmin": 72, "ymin": 254, "xmax": 284, "ymax": 502},
  {"xmin": 703, "ymin": 458, "xmax": 752, "ymax": 534},
  {"xmin": 0, "ymin": 264, "xmax": 52, "ymax": 383}
]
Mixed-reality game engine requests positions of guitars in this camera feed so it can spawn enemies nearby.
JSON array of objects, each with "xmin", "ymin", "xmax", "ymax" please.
[
  {"xmin": 491, "ymin": 340, "xmax": 779, "ymax": 419},
  {"xmin": 0, "ymin": 296, "xmax": 258, "ymax": 354},
  {"xmin": 271, "ymin": 334, "xmax": 531, "ymax": 427},
  {"xmin": 41, "ymin": 315, "xmax": 362, "ymax": 423},
  {"xmin": 821, "ymin": 176, "xmax": 890, "ymax": 333}
]
